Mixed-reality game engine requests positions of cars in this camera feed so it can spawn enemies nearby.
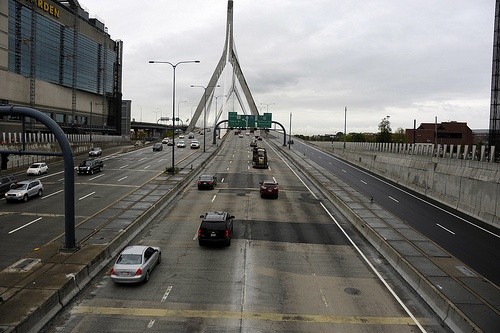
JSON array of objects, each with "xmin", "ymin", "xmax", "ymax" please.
[
  {"xmin": 111, "ymin": 244, "xmax": 163, "ymax": 287},
  {"xmin": 26, "ymin": 162, "xmax": 49, "ymax": 176},
  {"xmin": 88, "ymin": 147, "xmax": 102, "ymax": 157},
  {"xmin": 152, "ymin": 127, "xmax": 262, "ymax": 152},
  {"xmin": 198, "ymin": 174, "xmax": 217, "ymax": 191}
]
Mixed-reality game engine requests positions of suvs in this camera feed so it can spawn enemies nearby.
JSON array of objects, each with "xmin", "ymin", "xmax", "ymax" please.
[
  {"xmin": 76, "ymin": 157, "xmax": 103, "ymax": 176},
  {"xmin": 196, "ymin": 211, "xmax": 236, "ymax": 251},
  {"xmin": 5, "ymin": 179, "xmax": 44, "ymax": 204},
  {"xmin": 259, "ymin": 181, "xmax": 279, "ymax": 200}
]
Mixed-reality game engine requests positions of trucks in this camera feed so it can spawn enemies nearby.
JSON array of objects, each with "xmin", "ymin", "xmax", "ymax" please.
[{"xmin": 251, "ymin": 147, "xmax": 269, "ymax": 169}]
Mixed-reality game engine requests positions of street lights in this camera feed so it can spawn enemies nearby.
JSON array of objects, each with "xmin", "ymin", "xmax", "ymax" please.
[
  {"xmin": 190, "ymin": 84, "xmax": 221, "ymax": 153},
  {"xmin": 149, "ymin": 60, "xmax": 201, "ymax": 173}
]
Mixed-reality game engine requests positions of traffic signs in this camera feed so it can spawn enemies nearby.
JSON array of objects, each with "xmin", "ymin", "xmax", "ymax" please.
[{"xmin": 228, "ymin": 111, "xmax": 272, "ymax": 128}]
